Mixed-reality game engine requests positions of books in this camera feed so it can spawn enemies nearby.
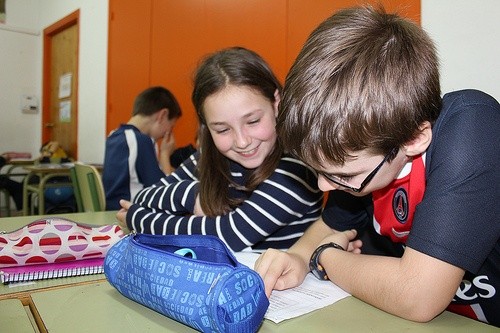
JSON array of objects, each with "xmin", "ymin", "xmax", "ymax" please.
[
  {"xmin": 232, "ymin": 247, "xmax": 352, "ymax": 323},
  {"xmin": 0, "ymin": 258, "xmax": 105, "ymax": 285}
]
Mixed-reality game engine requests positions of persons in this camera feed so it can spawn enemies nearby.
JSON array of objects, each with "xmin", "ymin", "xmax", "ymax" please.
[
  {"xmin": 115, "ymin": 46, "xmax": 325, "ymax": 252},
  {"xmin": 102, "ymin": 86, "xmax": 182, "ymax": 211},
  {"xmin": 254, "ymin": 2, "xmax": 500, "ymax": 332}
]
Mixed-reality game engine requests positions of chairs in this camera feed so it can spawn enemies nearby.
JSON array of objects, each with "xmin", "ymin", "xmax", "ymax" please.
[{"xmin": 1, "ymin": 156, "xmax": 108, "ymax": 217}]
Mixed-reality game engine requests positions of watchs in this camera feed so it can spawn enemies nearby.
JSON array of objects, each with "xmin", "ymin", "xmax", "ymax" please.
[{"xmin": 309, "ymin": 241, "xmax": 345, "ymax": 281}]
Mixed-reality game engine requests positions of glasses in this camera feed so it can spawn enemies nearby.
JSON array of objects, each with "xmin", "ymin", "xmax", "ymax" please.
[{"xmin": 318, "ymin": 145, "xmax": 399, "ymax": 193}]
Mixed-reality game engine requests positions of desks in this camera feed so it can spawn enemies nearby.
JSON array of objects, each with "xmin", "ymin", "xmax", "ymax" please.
[
  {"xmin": 0, "ymin": 160, "xmax": 84, "ymax": 217},
  {"xmin": 0, "ymin": 209, "xmax": 500, "ymax": 333}
]
